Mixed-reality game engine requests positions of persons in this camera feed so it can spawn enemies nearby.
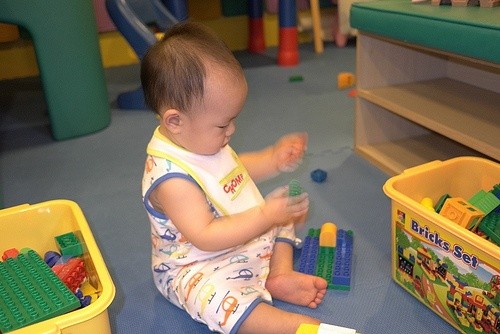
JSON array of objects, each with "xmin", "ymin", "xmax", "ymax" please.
[{"xmin": 137, "ymin": 23, "xmax": 330, "ymax": 334}]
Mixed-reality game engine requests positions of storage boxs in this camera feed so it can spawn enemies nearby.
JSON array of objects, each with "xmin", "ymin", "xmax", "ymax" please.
[
  {"xmin": 1, "ymin": 198, "xmax": 116, "ymax": 334},
  {"xmin": 382, "ymin": 156, "xmax": 500, "ymax": 334}
]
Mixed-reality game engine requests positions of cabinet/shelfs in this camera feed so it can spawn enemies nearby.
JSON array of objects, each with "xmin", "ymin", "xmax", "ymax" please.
[{"xmin": 352, "ymin": 29, "xmax": 500, "ymax": 181}]
